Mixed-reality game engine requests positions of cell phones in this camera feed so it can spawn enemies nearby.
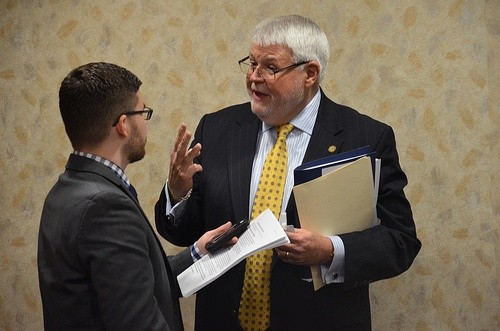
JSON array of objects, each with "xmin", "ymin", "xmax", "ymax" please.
[{"xmin": 205, "ymin": 218, "xmax": 249, "ymax": 254}]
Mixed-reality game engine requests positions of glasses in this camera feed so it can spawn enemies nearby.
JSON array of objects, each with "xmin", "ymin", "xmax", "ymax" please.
[
  {"xmin": 238, "ymin": 56, "xmax": 312, "ymax": 83},
  {"xmin": 112, "ymin": 106, "xmax": 154, "ymax": 127}
]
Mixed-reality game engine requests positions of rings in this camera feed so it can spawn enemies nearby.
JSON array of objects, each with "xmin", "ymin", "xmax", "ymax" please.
[{"xmin": 285, "ymin": 252, "xmax": 289, "ymax": 260}]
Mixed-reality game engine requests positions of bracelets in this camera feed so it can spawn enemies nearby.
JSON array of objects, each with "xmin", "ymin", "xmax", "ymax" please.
[
  {"xmin": 167, "ymin": 181, "xmax": 193, "ymax": 201},
  {"xmin": 328, "ymin": 250, "xmax": 334, "ymax": 267}
]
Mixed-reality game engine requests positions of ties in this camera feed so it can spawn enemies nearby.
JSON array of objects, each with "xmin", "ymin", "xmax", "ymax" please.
[{"xmin": 237, "ymin": 124, "xmax": 294, "ymax": 330}]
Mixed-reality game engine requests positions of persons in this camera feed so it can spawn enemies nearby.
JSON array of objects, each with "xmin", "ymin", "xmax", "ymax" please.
[
  {"xmin": 154, "ymin": 14, "xmax": 422, "ymax": 330},
  {"xmin": 37, "ymin": 62, "xmax": 238, "ymax": 331}
]
